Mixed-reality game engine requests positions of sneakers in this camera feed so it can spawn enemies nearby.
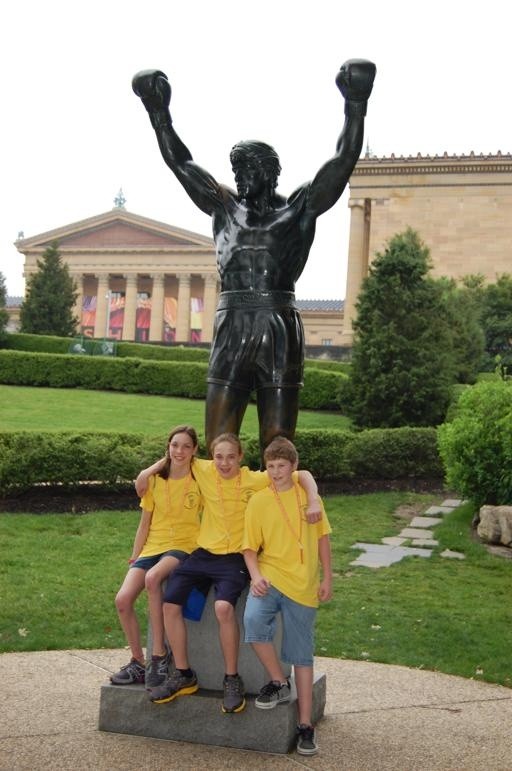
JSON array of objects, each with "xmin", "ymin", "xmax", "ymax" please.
[
  {"xmin": 294, "ymin": 722, "xmax": 319, "ymax": 756},
  {"xmin": 221, "ymin": 672, "xmax": 247, "ymax": 714},
  {"xmin": 109, "ymin": 644, "xmax": 199, "ymax": 705},
  {"xmin": 255, "ymin": 679, "xmax": 292, "ymax": 710}
]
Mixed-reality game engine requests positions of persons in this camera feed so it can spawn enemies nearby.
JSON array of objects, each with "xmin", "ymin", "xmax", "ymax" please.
[
  {"xmin": 241, "ymin": 437, "xmax": 336, "ymax": 758},
  {"xmin": 134, "ymin": 433, "xmax": 324, "ymax": 716},
  {"xmin": 107, "ymin": 426, "xmax": 206, "ymax": 692},
  {"xmin": 130, "ymin": 57, "xmax": 378, "ymax": 479}
]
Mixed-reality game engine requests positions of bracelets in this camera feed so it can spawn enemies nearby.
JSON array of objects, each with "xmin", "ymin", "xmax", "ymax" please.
[{"xmin": 126, "ymin": 560, "xmax": 136, "ymax": 565}]
